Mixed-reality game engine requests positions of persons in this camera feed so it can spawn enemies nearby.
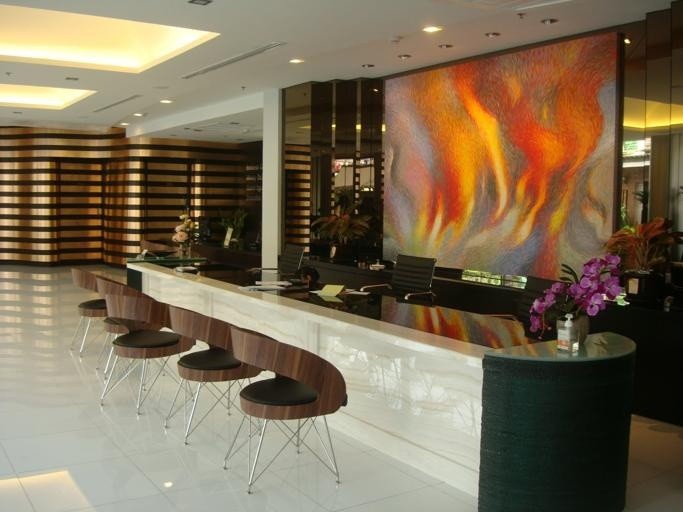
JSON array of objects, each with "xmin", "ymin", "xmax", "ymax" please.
[{"xmin": 296, "ymin": 267, "xmax": 325, "ymax": 287}]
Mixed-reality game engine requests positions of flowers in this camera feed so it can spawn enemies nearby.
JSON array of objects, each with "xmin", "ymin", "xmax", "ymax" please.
[
  {"xmin": 522, "ymin": 254, "xmax": 630, "ymax": 338},
  {"xmin": 171, "ymin": 213, "xmax": 203, "ymax": 243}
]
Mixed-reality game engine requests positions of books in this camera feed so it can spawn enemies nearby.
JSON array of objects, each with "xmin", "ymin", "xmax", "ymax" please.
[
  {"xmin": 176, "ymin": 266, "xmax": 198, "ymax": 272},
  {"xmin": 238, "ymin": 280, "xmax": 293, "ymax": 291},
  {"xmin": 309, "ymin": 284, "xmax": 346, "ymax": 303}
]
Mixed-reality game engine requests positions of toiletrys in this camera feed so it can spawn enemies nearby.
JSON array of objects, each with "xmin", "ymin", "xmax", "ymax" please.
[{"xmin": 556, "ymin": 313, "xmax": 579, "ymax": 353}]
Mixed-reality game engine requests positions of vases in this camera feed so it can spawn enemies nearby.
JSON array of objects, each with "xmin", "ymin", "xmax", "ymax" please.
[
  {"xmin": 555, "ymin": 315, "xmax": 592, "ymax": 354},
  {"xmin": 177, "ymin": 241, "xmax": 191, "ymax": 260}
]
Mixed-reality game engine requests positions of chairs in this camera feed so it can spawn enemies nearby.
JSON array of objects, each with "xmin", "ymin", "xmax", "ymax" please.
[
  {"xmin": 72, "ymin": 240, "xmax": 176, "ymax": 399},
  {"xmin": 220, "ymin": 324, "xmax": 350, "ymax": 494},
  {"xmin": 358, "ymin": 256, "xmax": 439, "ymax": 300},
  {"xmin": 99, "ymin": 293, "xmax": 196, "ymax": 416},
  {"xmin": 163, "ymin": 303, "xmax": 265, "ymax": 447},
  {"xmin": 254, "ymin": 243, "xmax": 305, "ymax": 276}
]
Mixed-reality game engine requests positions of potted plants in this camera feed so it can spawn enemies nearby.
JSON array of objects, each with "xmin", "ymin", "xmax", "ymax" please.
[
  {"xmin": 604, "ymin": 216, "xmax": 669, "ymax": 304},
  {"xmin": 310, "ymin": 204, "xmax": 372, "ymax": 261}
]
